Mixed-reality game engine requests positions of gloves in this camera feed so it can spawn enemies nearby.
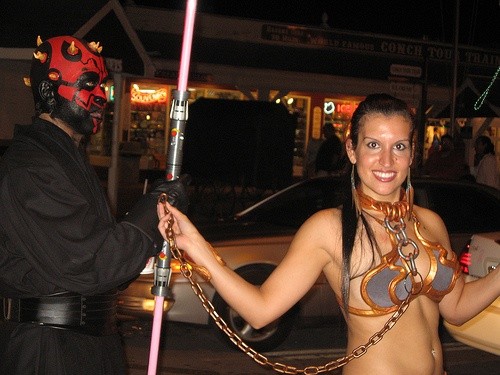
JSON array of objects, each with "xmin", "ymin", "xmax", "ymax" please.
[{"xmin": 118, "ymin": 173, "xmax": 195, "ymax": 252}]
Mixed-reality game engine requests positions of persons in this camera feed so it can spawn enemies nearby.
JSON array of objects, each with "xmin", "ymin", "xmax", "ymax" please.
[
  {"xmin": 157, "ymin": 95, "xmax": 500, "ymax": 375},
  {"xmin": 315, "ymin": 123, "xmax": 342, "ymax": 176},
  {"xmin": 447, "ymin": 136, "xmax": 465, "ymax": 157},
  {"xmin": 474, "ymin": 136, "xmax": 500, "ymax": 190},
  {"xmin": 0, "ymin": 36, "xmax": 189, "ymax": 375}
]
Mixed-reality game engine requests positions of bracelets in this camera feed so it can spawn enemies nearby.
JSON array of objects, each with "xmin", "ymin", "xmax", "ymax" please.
[{"xmin": 184, "ymin": 242, "xmax": 228, "ymax": 282}]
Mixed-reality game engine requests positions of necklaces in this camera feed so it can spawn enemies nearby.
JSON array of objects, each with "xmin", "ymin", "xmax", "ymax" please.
[{"xmin": 354, "ymin": 181, "xmax": 423, "ymax": 296}]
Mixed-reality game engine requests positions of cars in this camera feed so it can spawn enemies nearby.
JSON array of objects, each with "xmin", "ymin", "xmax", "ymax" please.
[{"xmin": 114, "ymin": 175, "xmax": 500, "ymax": 357}]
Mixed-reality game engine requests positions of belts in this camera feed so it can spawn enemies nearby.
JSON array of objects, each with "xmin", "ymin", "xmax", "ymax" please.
[{"xmin": 1, "ymin": 292, "xmax": 120, "ymax": 337}]
